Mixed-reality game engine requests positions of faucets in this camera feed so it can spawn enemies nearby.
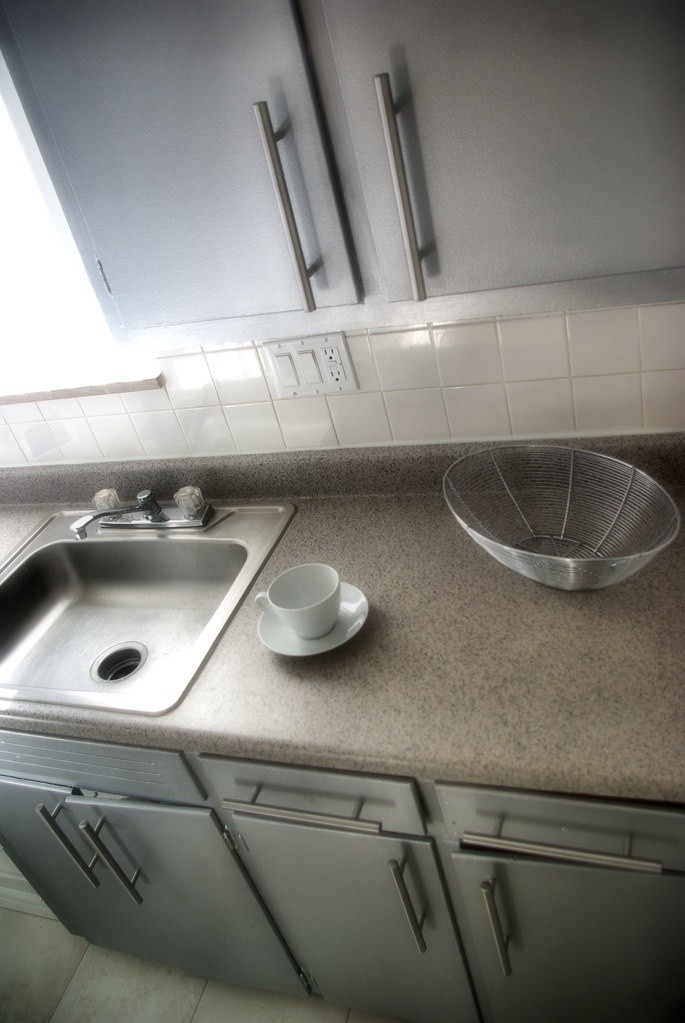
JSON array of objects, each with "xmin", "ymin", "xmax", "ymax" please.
[{"xmin": 69, "ymin": 488, "xmax": 164, "ymax": 541}]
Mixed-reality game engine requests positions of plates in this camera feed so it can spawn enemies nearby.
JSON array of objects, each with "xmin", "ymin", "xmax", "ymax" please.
[{"xmin": 259, "ymin": 581, "xmax": 369, "ymax": 657}]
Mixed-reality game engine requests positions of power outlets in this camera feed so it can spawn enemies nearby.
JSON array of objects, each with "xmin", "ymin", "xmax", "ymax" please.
[{"xmin": 263, "ymin": 332, "xmax": 361, "ymax": 401}]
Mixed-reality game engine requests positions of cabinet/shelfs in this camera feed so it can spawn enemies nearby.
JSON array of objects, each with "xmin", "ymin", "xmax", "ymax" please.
[
  {"xmin": 0, "ymin": 0, "xmax": 685, "ymax": 360},
  {"xmin": 0, "ymin": 731, "xmax": 685, "ymax": 1023}
]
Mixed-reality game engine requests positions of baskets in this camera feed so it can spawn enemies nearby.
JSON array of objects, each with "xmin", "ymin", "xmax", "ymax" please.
[{"xmin": 442, "ymin": 444, "xmax": 679, "ymax": 592}]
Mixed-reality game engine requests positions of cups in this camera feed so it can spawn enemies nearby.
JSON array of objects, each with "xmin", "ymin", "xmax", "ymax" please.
[{"xmin": 254, "ymin": 562, "xmax": 341, "ymax": 639}]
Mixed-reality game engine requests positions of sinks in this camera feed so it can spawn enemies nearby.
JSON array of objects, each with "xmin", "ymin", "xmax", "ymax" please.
[{"xmin": 0, "ymin": 501, "xmax": 298, "ymax": 717}]
